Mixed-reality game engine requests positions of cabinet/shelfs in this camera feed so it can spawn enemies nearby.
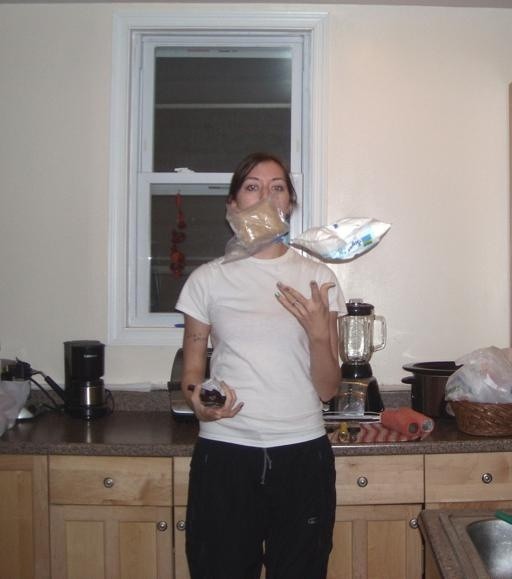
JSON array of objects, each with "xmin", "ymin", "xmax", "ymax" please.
[
  {"xmin": 314, "ymin": 455, "xmax": 424, "ymax": 575},
  {"xmin": 45, "ymin": 453, "xmax": 170, "ymax": 505},
  {"xmin": 1, "ymin": 455, "xmax": 49, "ymax": 575},
  {"xmin": 425, "ymin": 455, "xmax": 510, "ymax": 578},
  {"xmin": 49, "ymin": 506, "xmax": 172, "ymax": 579},
  {"xmin": 175, "ymin": 506, "xmax": 265, "ymax": 579}
]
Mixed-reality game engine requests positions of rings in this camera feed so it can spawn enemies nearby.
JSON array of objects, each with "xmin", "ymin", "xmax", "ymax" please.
[{"xmin": 292, "ymin": 300, "xmax": 299, "ymax": 306}]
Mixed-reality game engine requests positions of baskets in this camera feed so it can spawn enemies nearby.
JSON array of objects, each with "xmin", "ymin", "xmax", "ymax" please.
[{"xmin": 448, "ymin": 399, "xmax": 512, "ymax": 437}]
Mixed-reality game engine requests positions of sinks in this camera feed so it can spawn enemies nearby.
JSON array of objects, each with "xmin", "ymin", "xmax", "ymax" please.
[{"xmin": 438, "ymin": 510, "xmax": 512, "ymax": 579}]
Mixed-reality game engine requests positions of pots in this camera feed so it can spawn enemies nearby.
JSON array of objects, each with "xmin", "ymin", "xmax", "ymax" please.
[{"xmin": 402, "ymin": 361, "xmax": 468, "ymax": 419}]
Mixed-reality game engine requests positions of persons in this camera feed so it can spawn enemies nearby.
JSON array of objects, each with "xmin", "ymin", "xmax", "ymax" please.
[{"xmin": 172, "ymin": 149, "xmax": 348, "ymax": 578}]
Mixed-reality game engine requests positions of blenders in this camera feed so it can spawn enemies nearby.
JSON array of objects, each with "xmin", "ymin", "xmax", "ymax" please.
[{"xmin": 329, "ymin": 299, "xmax": 387, "ymax": 412}]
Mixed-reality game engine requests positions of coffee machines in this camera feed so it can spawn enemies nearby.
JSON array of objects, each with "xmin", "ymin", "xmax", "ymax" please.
[{"xmin": 62, "ymin": 340, "xmax": 114, "ymax": 420}]
